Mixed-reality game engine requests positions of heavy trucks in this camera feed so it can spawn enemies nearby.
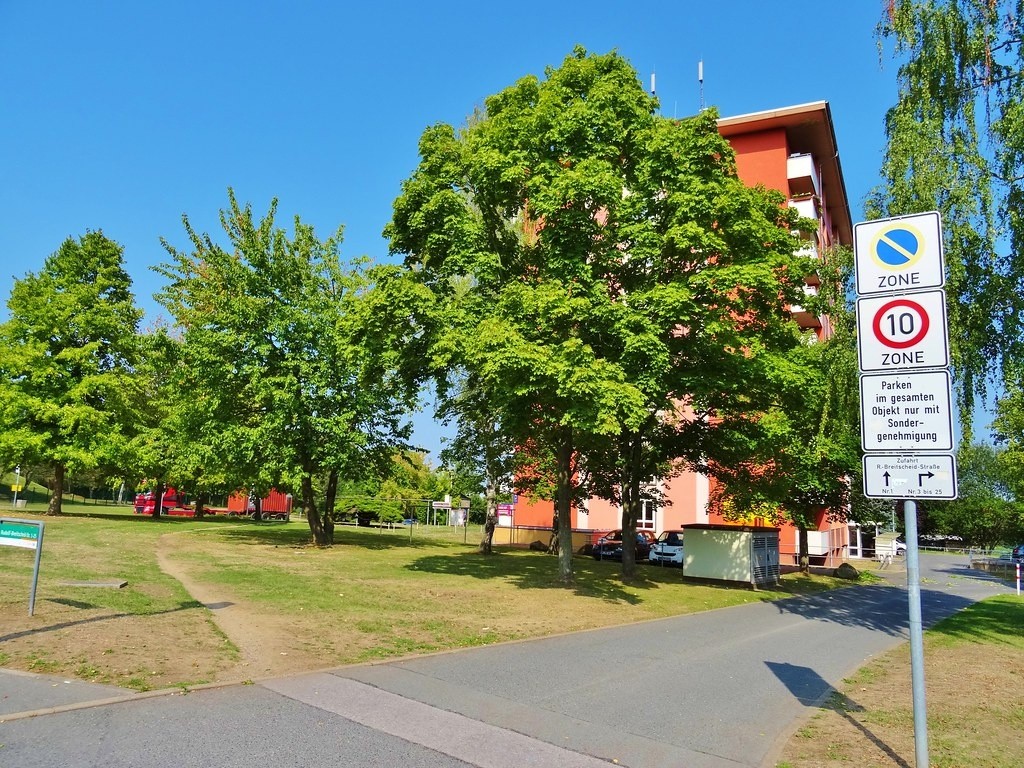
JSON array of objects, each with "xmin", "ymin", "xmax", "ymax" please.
[{"xmin": 133, "ymin": 476, "xmax": 293, "ymax": 522}]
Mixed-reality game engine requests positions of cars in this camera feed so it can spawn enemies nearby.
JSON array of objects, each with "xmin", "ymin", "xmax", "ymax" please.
[
  {"xmin": 1013, "ymin": 545, "xmax": 1024, "ymax": 564},
  {"xmin": 648, "ymin": 529, "xmax": 684, "ymax": 567},
  {"xmin": 896, "ymin": 539, "xmax": 907, "ymax": 556},
  {"xmin": 402, "ymin": 518, "xmax": 419, "ymax": 526},
  {"xmin": 591, "ymin": 529, "xmax": 658, "ymax": 562}
]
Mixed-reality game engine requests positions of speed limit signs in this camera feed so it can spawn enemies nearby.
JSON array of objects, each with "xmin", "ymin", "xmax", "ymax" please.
[{"xmin": 872, "ymin": 298, "xmax": 930, "ymax": 349}]
